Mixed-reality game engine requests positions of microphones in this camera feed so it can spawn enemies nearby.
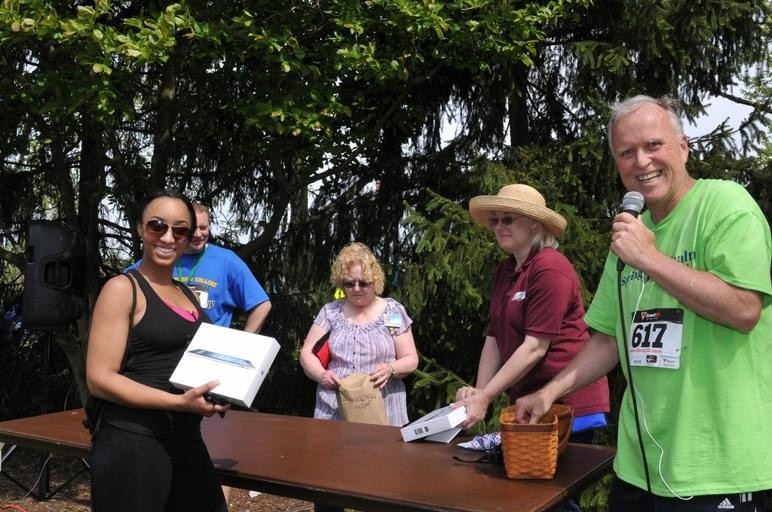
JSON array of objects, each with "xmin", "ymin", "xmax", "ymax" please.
[{"xmin": 616, "ymin": 191, "xmax": 646, "ymax": 273}]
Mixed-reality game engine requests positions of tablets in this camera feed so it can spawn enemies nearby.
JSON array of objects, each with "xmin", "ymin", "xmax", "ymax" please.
[{"xmin": 188, "ymin": 348, "xmax": 257, "ymax": 373}]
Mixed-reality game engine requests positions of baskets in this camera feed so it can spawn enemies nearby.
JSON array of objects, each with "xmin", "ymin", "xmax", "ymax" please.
[{"xmin": 499, "ymin": 403, "xmax": 574, "ymax": 482}]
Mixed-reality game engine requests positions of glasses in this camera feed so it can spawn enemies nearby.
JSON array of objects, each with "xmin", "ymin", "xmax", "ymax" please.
[
  {"xmin": 487, "ymin": 213, "xmax": 524, "ymax": 227},
  {"xmin": 342, "ymin": 280, "xmax": 373, "ymax": 288},
  {"xmin": 139, "ymin": 219, "xmax": 194, "ymax": 241}
]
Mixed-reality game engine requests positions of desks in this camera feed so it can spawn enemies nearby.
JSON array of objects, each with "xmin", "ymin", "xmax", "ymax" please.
[{"xmin": 0, "ymin": 408, "xmax": 617, "ymax": 512}]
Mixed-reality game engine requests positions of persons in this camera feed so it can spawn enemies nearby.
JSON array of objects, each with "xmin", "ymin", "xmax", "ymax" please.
[
  {"xmin": 450, "ymin": 211, "xmax": 609, "ymax": 447},
  {"xmin": 121, "ymin": 203, "xmax": 273, "ymax": 333},
  {"xmin": 81, "ymin": 190, "xmax": 232, "ymax": 512},
  {"xmin": 513, "ymin": 94, "xmax": 771, "ymax": 511},
  {"xmin": 298, "ymin": 240, "xmax": 418, "ymax": 512}
]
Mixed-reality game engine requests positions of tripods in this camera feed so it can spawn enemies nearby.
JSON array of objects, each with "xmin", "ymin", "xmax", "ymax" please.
[{"xmin": 0, "ymin": 355, "xmax": 93, "ymax": 502}]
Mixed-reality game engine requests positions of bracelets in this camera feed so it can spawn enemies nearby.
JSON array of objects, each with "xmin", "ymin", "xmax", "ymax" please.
[{"xmin": 389, "ymin": 364, "xmax": 394, "ymax": 380}]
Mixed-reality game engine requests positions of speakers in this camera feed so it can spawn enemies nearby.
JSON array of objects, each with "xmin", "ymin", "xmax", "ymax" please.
[{"xmin": 20, "ymin": 219, "xmax": 77, "ymax": 330}]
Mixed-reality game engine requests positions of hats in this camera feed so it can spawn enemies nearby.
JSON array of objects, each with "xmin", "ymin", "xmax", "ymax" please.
[{"xmin": 469, "ymin": 184, "xmax": 568, "ymax": 235}]
